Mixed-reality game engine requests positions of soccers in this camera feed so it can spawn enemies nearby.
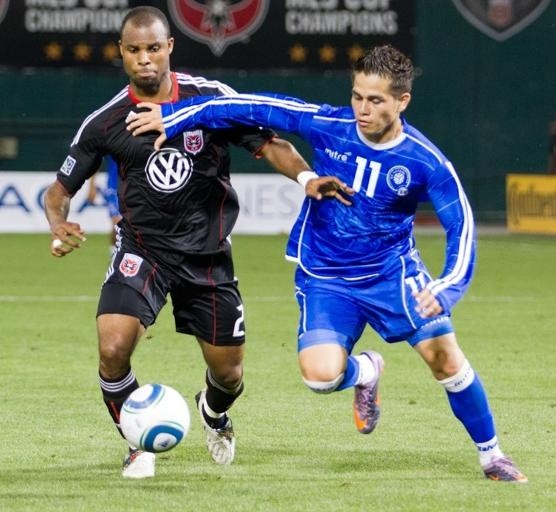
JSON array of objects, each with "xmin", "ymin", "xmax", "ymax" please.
[{"xmin": 120, "ymin": 383, "xmax": 190, "ymax": 453}]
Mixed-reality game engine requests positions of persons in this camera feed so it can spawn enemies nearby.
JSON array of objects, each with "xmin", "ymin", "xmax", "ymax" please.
[
  {"xmin": 124, "ymin": 42, "xmax": 529, "ymax": 484},
  {"xmin": 87, "ymin": 155, "xmax": 123, "ymax": 246},
  {"xmin": 42, "ymin": 5, "xmax": 357, "ymax": 480}
]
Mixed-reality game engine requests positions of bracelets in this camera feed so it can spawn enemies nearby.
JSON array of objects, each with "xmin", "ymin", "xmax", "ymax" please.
[{"xmin": 297, "ymin": 170, "xmax": 319, "ymax": 189}]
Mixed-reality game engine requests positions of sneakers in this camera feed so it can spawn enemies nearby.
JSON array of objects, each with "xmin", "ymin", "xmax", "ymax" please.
[
  {"xmin": 482, "ymin": 458, "xmax": 527, "ymax": 483},
  {"xmin": 121, "ymin": 447, "xmax": 155, "ymax": 478},
  {"xmin": 195, "ymin": 389, "xmax": 238, "ymax": 465},
  {"xmin": 353, "ymin": 351, "xmax": 384, "ymax": 433}
]
